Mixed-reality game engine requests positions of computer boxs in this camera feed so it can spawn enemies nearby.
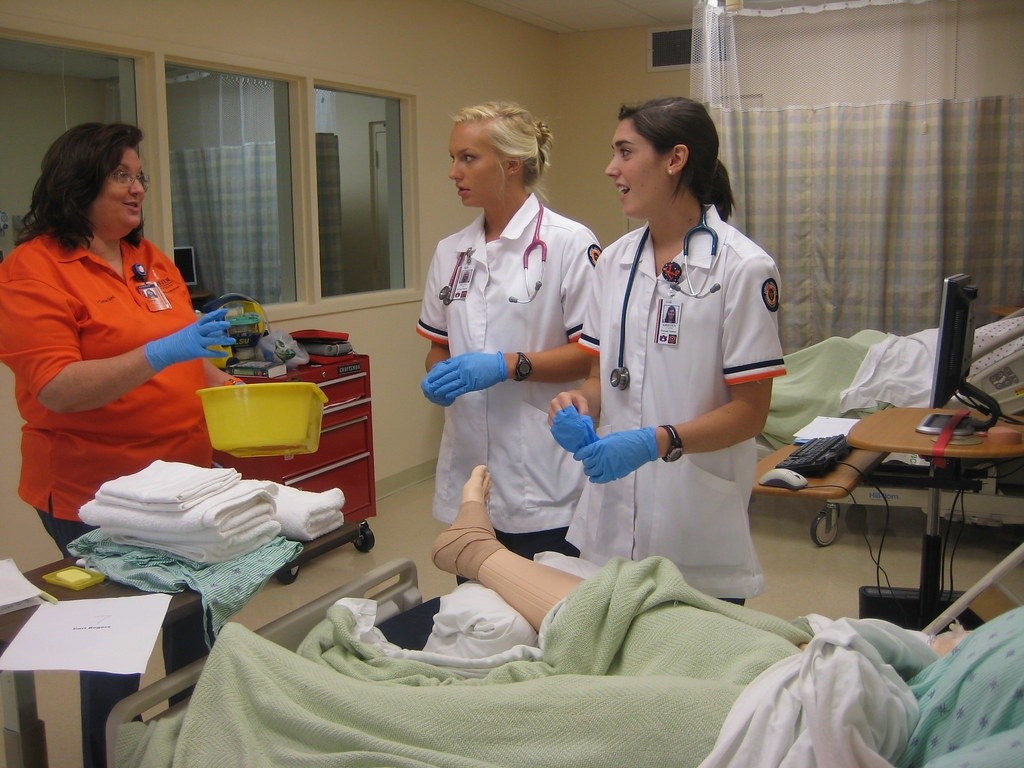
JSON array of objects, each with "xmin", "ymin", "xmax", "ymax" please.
[{"xmin": 858, "ymin": 585, "xmax": 969, "ymax": 636}]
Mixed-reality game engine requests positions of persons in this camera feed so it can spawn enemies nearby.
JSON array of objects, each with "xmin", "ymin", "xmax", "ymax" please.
[
  {"xmin": 416, "ymin": 102, "xmax": 603, "ymax": 584},
  {"xmin": 0, "ymin": 122, "xmax": 248, "ymax": 768},
  {"xmin": 548, "ymin": 95, "xmax": 786, "ymax": 606},
  {"xmin": 211, "ymin": 465, "xmax": 1024, "ymax": 768},
  {"xmin": 665, "ymin": 305, "xmax": 675, "ymax": 323},
  {"xmin": 461, "ymin": 271, "xmax": 470, "ymax": 283},
  {"xmin": 147, "ymin": 289, "xmax": 157, "ymax": 298}
]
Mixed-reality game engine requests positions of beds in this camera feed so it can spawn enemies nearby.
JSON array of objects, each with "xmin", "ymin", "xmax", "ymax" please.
[
  {"xmin": 758, "ymin": 307, "xmax": 1024, "ymax": 548},
  {"xmin": 102, "ymin": 539, "xmax": 1024, "ymax": 768}
]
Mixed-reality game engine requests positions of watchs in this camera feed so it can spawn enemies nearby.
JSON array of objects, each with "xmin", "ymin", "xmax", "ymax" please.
[
  {"xmin": 514, "ymin": 352, "xmax": 533, "ymax": 381},
  {"xmin": 660, "ymin": 425, "xmax": 682, "ymax": 462}
]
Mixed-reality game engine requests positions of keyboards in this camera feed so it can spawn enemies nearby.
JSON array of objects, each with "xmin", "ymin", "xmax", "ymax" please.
[{"xmin": 774, "ymin": 434, "xmax": 850, "ymax": 478}]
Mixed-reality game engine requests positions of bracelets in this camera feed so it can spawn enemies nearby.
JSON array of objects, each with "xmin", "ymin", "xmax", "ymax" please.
[{"xmin": 225, "ymin": 378, "xmax": 241, "ymax": 386}]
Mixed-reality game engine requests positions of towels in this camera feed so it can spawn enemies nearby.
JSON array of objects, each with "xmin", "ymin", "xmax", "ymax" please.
[
  {"xmin": 698, "ymin": 613, "xmax": 940, "ymax": 768},
  {"xmin": 75, "ymin": 458, "xmax": 346, "ymax": 565},
  {"xmin": 839, "ymin": 327, "xmax": 938, "ymax": 412}
]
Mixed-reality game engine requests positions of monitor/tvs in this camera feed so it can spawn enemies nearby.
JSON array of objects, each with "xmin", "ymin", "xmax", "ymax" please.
[
  {"xmin": 172, "ymin": 245, "xmax": 197, "ymax": 286},
  {"xmin": 915, "ymin": 272, "xmax": 1001, "ymax": 436}
]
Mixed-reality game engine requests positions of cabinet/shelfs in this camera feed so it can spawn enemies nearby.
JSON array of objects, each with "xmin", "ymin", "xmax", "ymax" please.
[{"xmin": 214, "ymin": 353, "xmax": 377, "ymax": 523}]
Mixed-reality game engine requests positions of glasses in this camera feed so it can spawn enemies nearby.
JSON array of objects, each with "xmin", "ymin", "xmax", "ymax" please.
[{"xmin": 108, "ymin": 171, "xmax": 150, "ymax": 192}]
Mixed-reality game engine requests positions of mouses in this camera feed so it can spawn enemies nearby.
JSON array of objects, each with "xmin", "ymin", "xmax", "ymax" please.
[{"xmin": 759, "ymin": 468, "xmax": 808, "ymax": 491}]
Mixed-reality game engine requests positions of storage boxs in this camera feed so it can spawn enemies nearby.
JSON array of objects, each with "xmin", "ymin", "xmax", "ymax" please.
[{"xmin": 199, "ymin": 380, "xmax": 330, "ymax": 459}]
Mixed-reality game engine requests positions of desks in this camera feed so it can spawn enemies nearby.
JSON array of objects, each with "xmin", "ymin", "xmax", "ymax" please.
[
  {"xmin": 0, "ymin": 522, "xmax": 365, "ymax": 768},
  {"xmin": 754, "ymin": 407, "xmax": 1024, "ymax": 635}
]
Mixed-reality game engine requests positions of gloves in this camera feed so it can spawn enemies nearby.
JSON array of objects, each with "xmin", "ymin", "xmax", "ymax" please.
[
  {"xmin": 145, "ymin": 308, "xmax": 235, "ymax": 372},
  {"xmin": 573, "ymin": 426, "xmax": 658, "ymax": 484},
  {"xmin": 421, "ymin": 363, "xmax": 455, "ymax": 407},
  {"xmin": 430, "ymin": 351, "xmax": 507, "ymax": 399},
  {"xmin": 551, "ymin": 406, "xmax": 599, "ymax": 454}
]
